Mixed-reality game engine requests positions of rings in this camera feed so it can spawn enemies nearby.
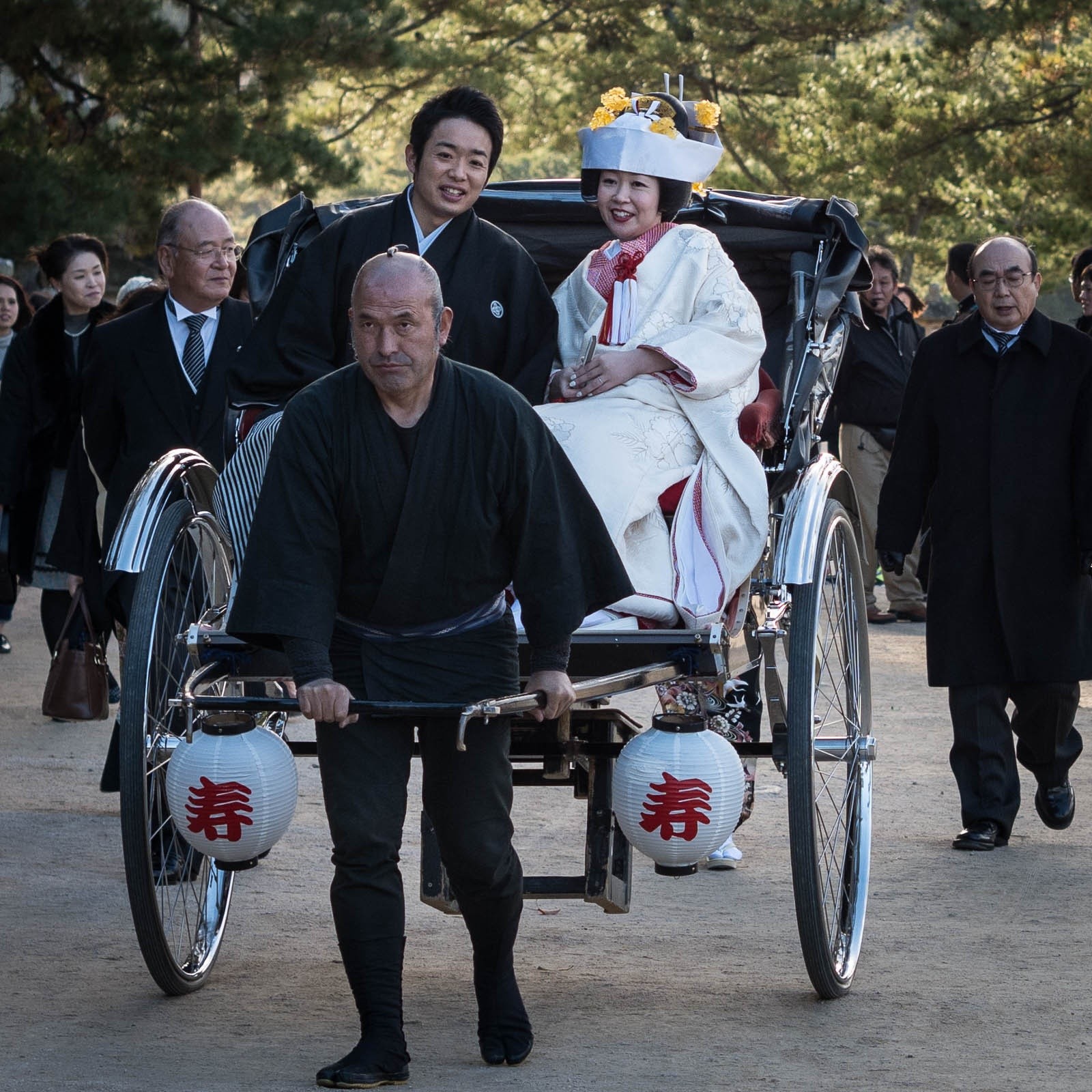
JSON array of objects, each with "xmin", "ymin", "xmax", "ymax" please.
[{"xmin": 597, "ymin": 376, "xmax": 605, "ymax": 384}]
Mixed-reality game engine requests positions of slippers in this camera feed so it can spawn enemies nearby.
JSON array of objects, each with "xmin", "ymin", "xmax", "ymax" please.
[{"xmin": 706, "ymin": 842, "xmax": 738, "ymax": 870}]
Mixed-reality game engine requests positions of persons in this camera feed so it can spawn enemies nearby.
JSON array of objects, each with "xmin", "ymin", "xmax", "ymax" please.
[
  {"xmin": 939, "ymin": 241, "xmax": 978, "ymax": 329},
  {"xmin": 1079, "ymin": 264, "xmax": 1092, "ymax": 316},
  {"xmin": 503, "ymin": 84, "xmax": 773, "ymax": 632},
  {"xmin": 892, "ymin": 280, "xmax": 926, "ymax": 345},
  {"xmin": 228, "ymin": 248, "xmax": 636, "ymax": 1089},
  {"xmin": 3, "ymin": 234, "xmax": 121, "ymax": 704},
  {"xmin": 80, "ymin": 200, "xmax": 258, "ymax": 885},
  {"xmin": 832, "ymin": 245, "xmax": 928, "ymax": 623},
  {"xmin": 872, "ymin": 235, "xmax": 1090, "ymax": 852},
  {"xmin": 1, "ymin": 275, "xmax": 32, "ymax": 373},
  {"xmin": 1068, "ymin": 244, "xmax": 1092, "ymax": 332},
  {"xmin": 210, "ymin": 84, "xmax": 562, "ymax": 636}
]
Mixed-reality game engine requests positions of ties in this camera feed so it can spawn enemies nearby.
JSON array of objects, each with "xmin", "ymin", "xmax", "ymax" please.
[
  {"xmin": 165, "ymin": 293, "xmax": 220, "ymax": 390},
  {"xmin": 980, "ymin": 316, "xmax": 1028, "ymax": 356}
]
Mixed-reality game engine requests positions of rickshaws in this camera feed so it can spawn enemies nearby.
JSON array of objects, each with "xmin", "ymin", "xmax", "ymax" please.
[{"xmin": 102, "ymin": 176, "xmax": 880, "ymax": 998}]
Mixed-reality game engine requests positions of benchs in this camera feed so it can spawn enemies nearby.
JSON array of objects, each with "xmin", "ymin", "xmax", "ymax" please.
[{"xmin": 309, "ymin": 228, "xmax": 808, "ymax": 494}]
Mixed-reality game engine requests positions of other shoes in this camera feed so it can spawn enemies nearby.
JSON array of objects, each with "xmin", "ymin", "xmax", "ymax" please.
[
  {"xmin": 315, "ymin": 936, "xmax": 412, "ymax": 1090},
  {"xmin": 867, "ymin": 605, "xmax": 897, "ymax": 625},
  {"xmin": 887, "ymin": 602, "xmax": 927, "ymax": 623},
  {"xmin": 0, "ymin": 634, "xmax": 12, "ymax": 654},
  {"xmin": 462, "ymin": 899, "xmax": 535, "ymax": 1066}
]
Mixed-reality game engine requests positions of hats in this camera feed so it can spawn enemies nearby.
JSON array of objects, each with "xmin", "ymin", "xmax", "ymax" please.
[{"xmin": 579, "ymin": 73, "xmax": 724, "ymax": 185}]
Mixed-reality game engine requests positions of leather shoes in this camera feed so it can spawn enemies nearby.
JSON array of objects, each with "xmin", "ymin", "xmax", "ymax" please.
[
  {"xmin": 952, "ymin": 819, "xmax": 1008, "ymax": 851},
  {"xmin": 1035, "ymin": 771, "xmax": 1075, "ymax": 830}
]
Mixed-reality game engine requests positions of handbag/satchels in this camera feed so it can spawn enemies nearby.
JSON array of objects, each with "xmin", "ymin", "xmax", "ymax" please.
[{"xmin": 41, "ymin": 585, "xmax": 110, "ymax": 723}]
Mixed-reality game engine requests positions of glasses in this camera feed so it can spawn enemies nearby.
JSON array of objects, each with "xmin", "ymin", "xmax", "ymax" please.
[
  {"xmin": 971, "ymin": 270, "xmax": 1036, "ymax": 291},
  {"xmin": 164, "ymin": 243, "xmax": 242, "ymax": 266}
]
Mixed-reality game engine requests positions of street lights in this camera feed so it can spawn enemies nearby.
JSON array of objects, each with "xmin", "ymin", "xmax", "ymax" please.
[{"xmin": 141, "ymin": 1, "xmax": 201, "ymax": 200}]
